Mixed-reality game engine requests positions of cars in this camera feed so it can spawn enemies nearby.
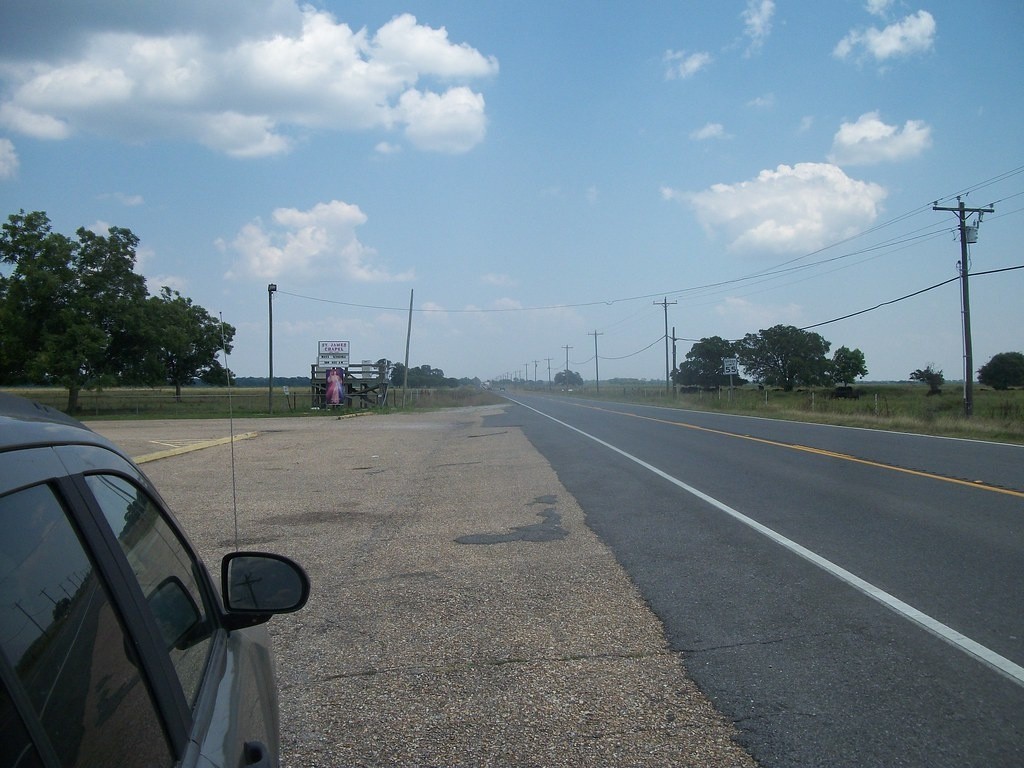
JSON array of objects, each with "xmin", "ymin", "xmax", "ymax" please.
[{"xmin": 0, "ymin": 389, "xmax": 310, "ymax": 768}]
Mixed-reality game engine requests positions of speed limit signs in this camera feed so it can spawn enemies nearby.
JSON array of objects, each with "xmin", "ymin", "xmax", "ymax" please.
[{"xmin": 724, "ymin": 358, "xmax": 737, "ymax": 375}]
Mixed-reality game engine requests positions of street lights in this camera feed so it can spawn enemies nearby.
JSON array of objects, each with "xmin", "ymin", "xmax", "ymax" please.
[{"xmin": 268, "ymin": 283, "xmax": 278, "ymax": 415}]
